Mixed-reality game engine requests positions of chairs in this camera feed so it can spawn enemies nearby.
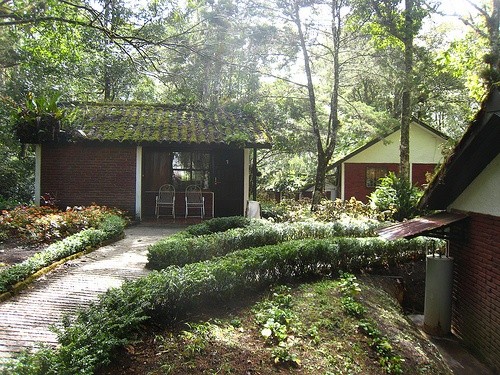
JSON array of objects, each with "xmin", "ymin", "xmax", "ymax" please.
[
  {"xmin": 155, "ymin": 183, "xmax": 176, "ymax": 220},
  {"xmin": 184, "ymin": 185, "xmax": 206, "ymax": 220}
]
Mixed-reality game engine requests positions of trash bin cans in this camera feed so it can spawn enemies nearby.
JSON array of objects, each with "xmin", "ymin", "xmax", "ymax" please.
[{"xmin": 245, "ymin": 200, "xmax": 262, "ymax": 220}]
